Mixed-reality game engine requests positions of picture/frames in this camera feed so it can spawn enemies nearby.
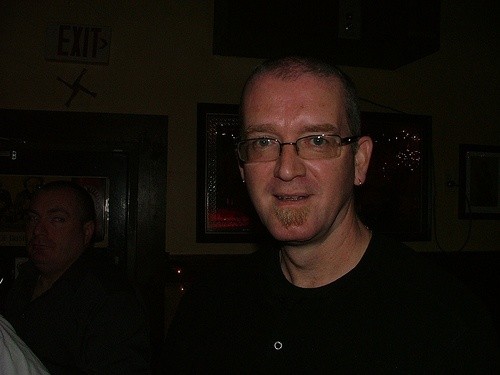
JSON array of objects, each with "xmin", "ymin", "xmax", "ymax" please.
[
  {"xmin": 196, "ymin": 102, "xmax": 435, "ymax": 248},
  {"xmin": 458, "ymin": 144, "xmax": 500, "ymax": 221}
]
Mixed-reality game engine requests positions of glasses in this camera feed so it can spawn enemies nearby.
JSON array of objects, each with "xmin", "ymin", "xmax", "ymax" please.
[{"xmin": 236, "ymin": 133, "xmax": 354, "ymax": 163}]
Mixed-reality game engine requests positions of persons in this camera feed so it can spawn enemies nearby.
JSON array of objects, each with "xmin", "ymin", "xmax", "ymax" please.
[
  {"xmin": 1, "ymin": 181, "xmax": 158, "ymax": 374},
  {"xmin": 145, "ymin": 52, "xmax": 500, "ymax": 375}
]
